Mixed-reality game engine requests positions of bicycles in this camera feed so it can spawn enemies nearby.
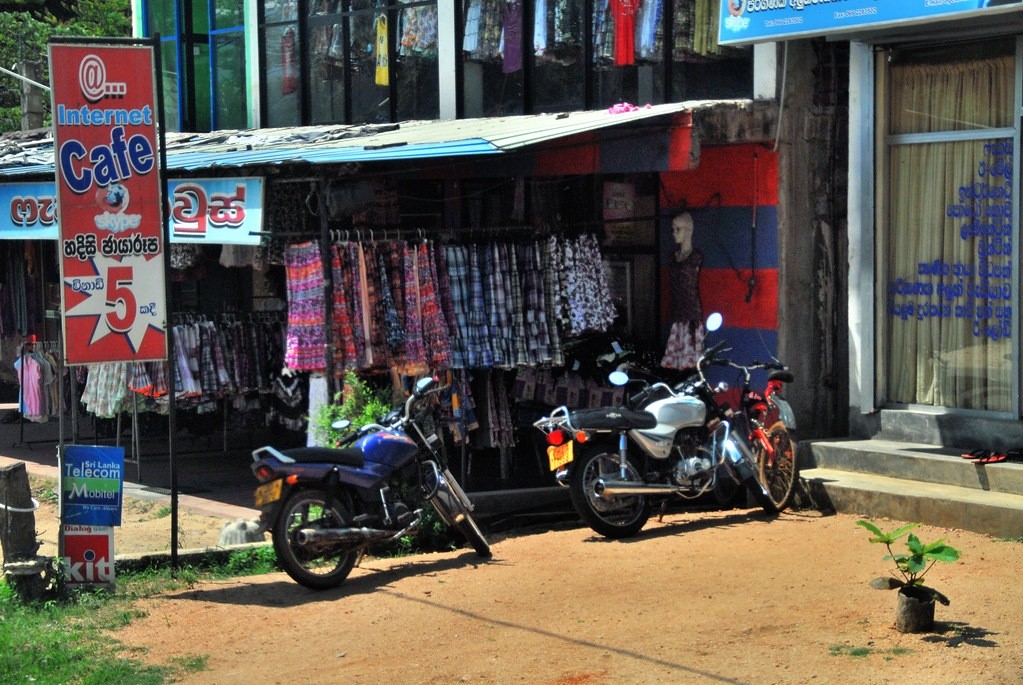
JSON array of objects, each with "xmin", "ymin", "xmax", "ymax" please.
[{"xmin": 710, "ymin": 357, "xmax": 801, "ymax": 514}]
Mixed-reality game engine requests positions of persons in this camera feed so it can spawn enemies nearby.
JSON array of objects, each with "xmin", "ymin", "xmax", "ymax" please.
[{"xmin": 658, "ymin": 213, "xmax": 710, "ymax": 370}]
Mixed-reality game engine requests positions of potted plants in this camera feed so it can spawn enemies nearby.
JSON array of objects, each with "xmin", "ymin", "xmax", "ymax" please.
[{"xmin": 857, "ymin": 516, "xmax": 961, "ymax": 635}]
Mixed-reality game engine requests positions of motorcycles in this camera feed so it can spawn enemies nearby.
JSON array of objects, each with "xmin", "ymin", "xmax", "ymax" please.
[
  {"xmin": 528, "ymin": 312, "xmax": 775, "ymax": 537},
  {"xmin": 250, "ymin": 372, "xmax": 494, "ymax": 592}
]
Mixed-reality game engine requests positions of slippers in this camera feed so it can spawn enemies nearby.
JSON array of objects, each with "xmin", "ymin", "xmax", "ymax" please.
[
  {"xmin": 971, "ymin": 450, "xmax": 1009, "ymax": 464},
  {"xmin": 961, "ymin": 446, "xmax": 989, "ymax": 458}
]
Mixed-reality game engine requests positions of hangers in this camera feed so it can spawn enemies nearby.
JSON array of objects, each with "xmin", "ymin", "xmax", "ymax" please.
[{"xmin": 20, "ymin": 209, "xmax": 601, "ymax": 368}]
